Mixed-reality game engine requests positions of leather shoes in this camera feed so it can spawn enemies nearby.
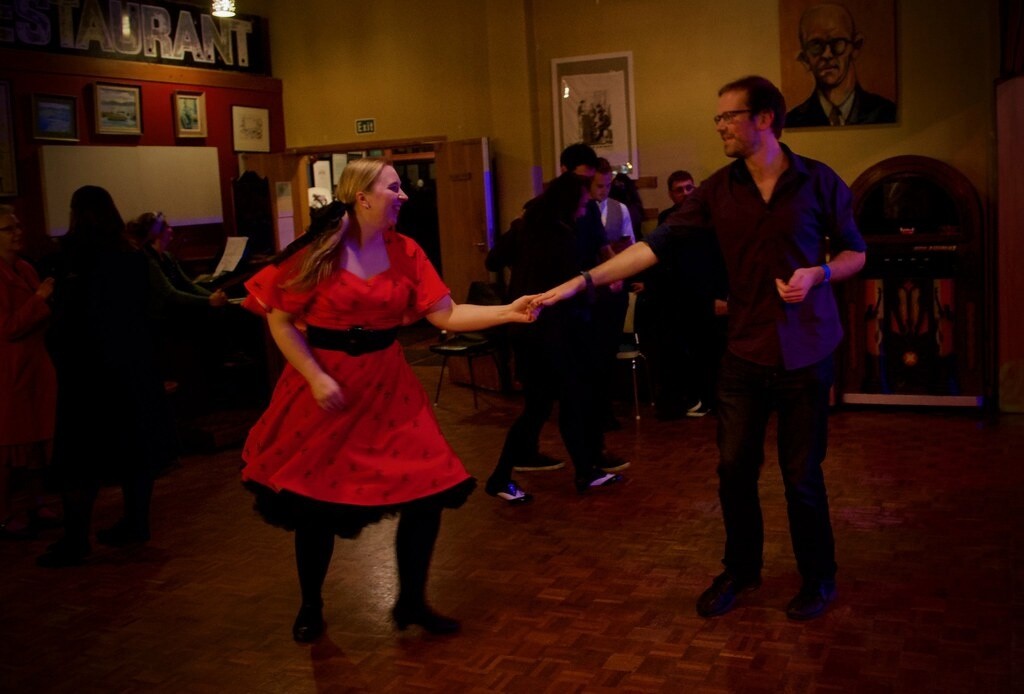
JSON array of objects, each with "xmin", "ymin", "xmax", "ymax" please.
[
  {"xmin": 786, "ymin": 583, "xmax": 835, "ymax": 621},
  {"xmin": 485, "ymin": 478, "xmax": 533, "ymax": 505},
  {"xmin": 575, "ymin": 468, "xmax": 622, "ymax": 495},
  {"xmin": 696, "ymin": 570, "xmax": 762, "ymax": 617}
]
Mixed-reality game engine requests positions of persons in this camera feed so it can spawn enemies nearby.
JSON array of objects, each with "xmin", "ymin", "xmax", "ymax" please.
[
  {"xmin": 527, "ymin": 75, "xmax": 867, "ymax": 622},
  {"xmin": 483, "ymin": 144, "xmax": 730, "ymax": 506},
  {"xmin": 239, "ymin": 159, "xmax": 545, "ymax": 645},
  {"xmin": 0, "ymin": 185, "xmax": 227, "ymax": 570}
]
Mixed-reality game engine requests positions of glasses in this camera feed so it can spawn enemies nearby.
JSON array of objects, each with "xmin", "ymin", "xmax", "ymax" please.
[{"xmin": 713, "ymin": 109, "xmax": 762, "ymax": 124}]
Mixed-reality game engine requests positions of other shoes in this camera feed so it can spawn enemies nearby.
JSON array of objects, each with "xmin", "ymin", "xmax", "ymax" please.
[
  {"xmin": 597, "ymin": 454, "xmax": 631, "ymax": 472},
  {"xmin": 98, "ymin": 518, "xmax": 152, "ymax": 548},
  {"xmin": 514, "ymin": 453, "xmax": 566, "ymax": 471},
  {"xmin": 685, "ymin": 400, "xmax": 711, "ymax": 416},
  {"xmin": 44, "ymin": 540, "xmax": 92, "ymax": 559}
]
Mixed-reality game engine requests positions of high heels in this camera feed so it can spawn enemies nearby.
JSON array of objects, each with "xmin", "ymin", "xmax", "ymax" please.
[
  {"xmin": 292, "ymin": 603, "xmax": 324, "ymax": 642},
  {"xmin": 391, "ymin": 600, "xmax": 460, "ymax": 636}
]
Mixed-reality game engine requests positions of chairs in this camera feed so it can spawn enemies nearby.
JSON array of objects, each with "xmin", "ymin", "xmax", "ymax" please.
[{"xmin": 428, "ymin": 282, "xmax": 504, "ymax": 409}]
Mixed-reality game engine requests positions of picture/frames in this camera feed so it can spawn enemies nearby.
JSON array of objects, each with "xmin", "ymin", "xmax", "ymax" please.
[
  {"xmin": 778, "ymin": 0, "xmax": 900, "ymax": 133},
  {"xmin": 31, "ymin": 92, "xmax": 80, "ymax": 142},
  {"xmin": 0, "ymin": 78, "xmax": 18, "ymax": 196},
  {"xmin": 231, "ymin": 104, "xmax": 272, "ymax": 154},
  {"xmin": 91, "ymin": 79, "xmax": 145, "ymax": 136},
  {"xmin": 172, "ymin": 89, "xmax": 207, "ymax": 137},
  {"xmin": 550, "ymin": 50, "xmax": 640, "ymax": 180}
]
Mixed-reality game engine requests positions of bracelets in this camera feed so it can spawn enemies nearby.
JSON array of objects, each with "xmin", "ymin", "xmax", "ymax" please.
[
  {"xmin": 812, "ymin": 263, "xmax": 831, "ymax": 291},
  {"xmin": 580, "ymin": 270, "xmax": 594, "ymax": 291}
]
